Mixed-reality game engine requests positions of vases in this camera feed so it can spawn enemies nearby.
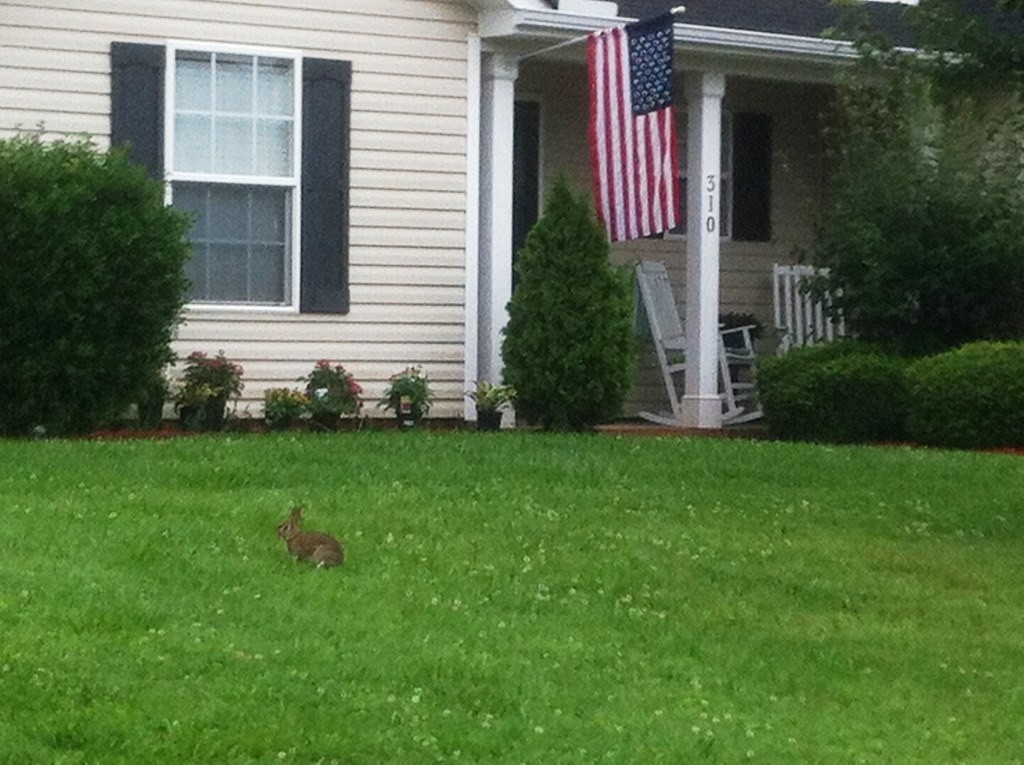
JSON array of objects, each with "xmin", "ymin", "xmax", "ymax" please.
[
  {"xmin": 308, "ymin": 411, "xmax": 342, "ymax": 432},
  {"xmin": 179, "ymin": 400, "xmax": 226, "ymax": 434},
  {"xmin": 396, "ymin": 410, "xmax": 423, "ymax": 434}
]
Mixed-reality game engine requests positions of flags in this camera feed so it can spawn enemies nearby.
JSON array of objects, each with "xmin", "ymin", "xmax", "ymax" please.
[{"xmin": 585, "ymin": 12, "xmax": 682, "ymax": 244}]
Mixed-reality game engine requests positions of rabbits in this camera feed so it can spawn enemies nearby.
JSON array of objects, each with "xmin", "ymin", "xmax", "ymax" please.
[{"xmin": 276, "ymin": 507, "xmax": 345, "ymax": 571}]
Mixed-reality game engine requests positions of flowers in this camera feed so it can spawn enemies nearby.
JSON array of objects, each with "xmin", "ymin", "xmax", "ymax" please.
[
  {"xmin": 296, "ymin": 360, "xmax": 364, "ymax": 418},
  {"xmin": 376, "ymin": 363, "xmax": 436, "ymax": 416},
  {"xmin": 169, "ymin": 350, "xmax": 247, "ymax": 410}
]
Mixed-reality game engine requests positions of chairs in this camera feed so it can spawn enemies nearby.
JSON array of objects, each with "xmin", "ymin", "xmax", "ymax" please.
[
  {"xmin": 772, "ymin": 263, "xmax": 859, "ymax": 357},
  {"xmin": 636, "ymin": 260, "xmax": 765, "ymax": 425}
]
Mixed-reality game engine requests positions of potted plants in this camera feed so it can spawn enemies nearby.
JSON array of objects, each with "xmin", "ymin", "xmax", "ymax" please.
[
  {"xmin": 463, "ymin": 380, "xmax": 518, "ymax": 431},
  {"xmin": 260, "ymin": 387, "xmax": 308, "ymax": 430},
  {"xmin": 719, "ymin": 312, "xmax": 764, "ymax": 408}
]
